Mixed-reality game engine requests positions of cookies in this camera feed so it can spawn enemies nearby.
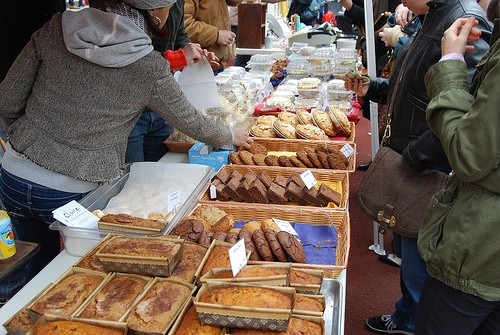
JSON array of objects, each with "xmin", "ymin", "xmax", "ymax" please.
[
  {"xmin": 231, "ymin": 142, "xmax": 349, "ymax": 171},
  {"xmin": 167, "ymin": 205, "xmax": 306, "ymax": 264}
]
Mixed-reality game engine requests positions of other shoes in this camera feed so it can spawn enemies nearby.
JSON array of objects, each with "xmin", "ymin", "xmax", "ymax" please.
[{"xmin": 358, "ymin": 161, "xmax": 372, "ymax": 171}]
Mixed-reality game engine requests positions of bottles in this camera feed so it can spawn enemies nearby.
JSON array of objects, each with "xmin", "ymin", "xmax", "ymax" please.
[{"xmin": 177, "ymin": 50, "xmax": 222, "ymax": 120}]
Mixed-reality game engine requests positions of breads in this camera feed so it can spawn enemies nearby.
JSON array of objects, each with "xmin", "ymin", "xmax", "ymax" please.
[
  {"xmin": 9, "ymin": 237, "xmax": 325, "ymax": 335},
  {"xmin": 209, "ymin": 168, "xmax": 342, "ymax": 208},
  {"xmin": 97, "ymin": 212, "xmax": 168, "ymax": 234},
  {"xmin": 249, "ymin": 106, "xmax": 352, "ymax": 141}
]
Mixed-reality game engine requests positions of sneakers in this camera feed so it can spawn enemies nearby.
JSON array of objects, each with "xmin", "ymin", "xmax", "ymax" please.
[{"xmin": 366, "ymin": 315, "xmax": 414, "ymax": 334}]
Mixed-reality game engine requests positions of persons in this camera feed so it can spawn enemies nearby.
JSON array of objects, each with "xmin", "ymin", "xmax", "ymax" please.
[
  {"xmin": 183, "ymin": 0, "xmax": 237, "ymax": 77},
  {"xmin": 227, "ymin": 0, "xmax": 286, "ymax": 68},
  {"xmin": 412, "ymin": 17, "xmax": 499, "ymax": 335},
  {"xmin": 125, "ymin": 0, "xmax": 221, "ymax": 165},
  {"xmin": 341, "ymin": 0, "xmax": 492, "ymax": 332},
  {"xmin": 287, "ymin": 0, "xmax": 500, "ymax": 169},
  {"xmin": 0, "ymin": 0, "xmax": 254, "ymax": 265},
  {"xmin": 0, "ymin": 0, "xmax": 89, "ymax": 164}
]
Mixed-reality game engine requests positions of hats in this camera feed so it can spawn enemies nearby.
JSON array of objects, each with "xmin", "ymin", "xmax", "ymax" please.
[{"xmin": 126, "ymin": 0, "xmax": 178, "ymax": 10}]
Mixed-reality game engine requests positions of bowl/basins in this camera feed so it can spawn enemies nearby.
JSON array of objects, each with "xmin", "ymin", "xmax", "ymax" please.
[
  {"xmin": 217, "ymin": 52, "xmax": 285, "ymax": 133},
  {"xmin": 266, "ymin": 42, "xmax": 356, "ymax": 113}
]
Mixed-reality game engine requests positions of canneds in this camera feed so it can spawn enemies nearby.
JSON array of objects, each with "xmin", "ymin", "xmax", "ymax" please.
[{"xmin": 0, "ymin": 210, "xmax": 16, "ymax": 260}]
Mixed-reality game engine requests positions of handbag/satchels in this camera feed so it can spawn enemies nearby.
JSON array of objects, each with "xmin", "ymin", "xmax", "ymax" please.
[{"xmin": 358, "ymin": 146, "xmax": 451, "ymax": 239}]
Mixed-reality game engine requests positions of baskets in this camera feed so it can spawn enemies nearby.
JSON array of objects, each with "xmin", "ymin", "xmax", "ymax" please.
[{"xmin": 187, "ymin": 136, "xmax": 356, "ymax": 279}]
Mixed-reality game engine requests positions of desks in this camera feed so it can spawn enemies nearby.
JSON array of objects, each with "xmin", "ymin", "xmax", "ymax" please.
[{"xmin": 0, "ymin": 240, "xmax": 40, "ymax": 283}]
